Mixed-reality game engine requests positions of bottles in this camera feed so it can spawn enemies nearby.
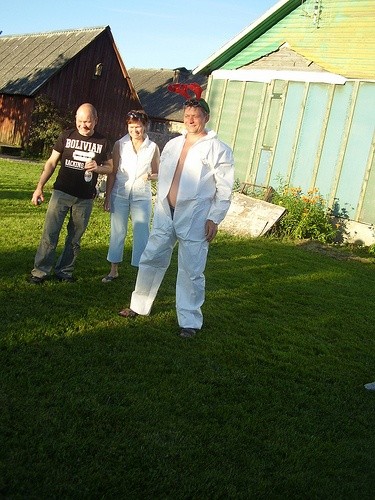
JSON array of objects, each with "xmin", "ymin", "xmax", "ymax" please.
[{"xmin": 84, "ymin": 158, "xmax": 92, "ymax": 182}]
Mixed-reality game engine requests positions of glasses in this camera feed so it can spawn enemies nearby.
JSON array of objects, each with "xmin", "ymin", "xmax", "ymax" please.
[
  {"xmin": 184, "ymin": 101, "xmax": 207, "ymax": 114},
  {"xmin": 127, "ymin": 112, "xmax": 146, "ymax": 119}
]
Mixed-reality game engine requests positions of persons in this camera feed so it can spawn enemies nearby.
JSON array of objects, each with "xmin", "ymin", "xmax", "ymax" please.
[
  {"xmin": 100, "ymin": 110, "xmax": 162, "ymax": 285},
  {"xmin": 25, "ymin": 103, "xmax": 114, "ymax": 285},
  {"xmin": 117, "ymin": 97, "xmax": 234, "ymax": 339}
]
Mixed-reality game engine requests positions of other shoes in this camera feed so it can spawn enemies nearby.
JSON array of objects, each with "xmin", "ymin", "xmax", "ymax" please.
[
  {"xmin": 25, "ymin": 272, "xmax": 48, "ymax": 282},
  {"xmin": 58, "ymin": 272, "xmax": 75, "ymax": 282}
]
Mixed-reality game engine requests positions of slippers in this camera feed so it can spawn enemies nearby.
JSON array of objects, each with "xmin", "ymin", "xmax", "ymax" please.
[
  {"xmin": 117, "ymin": 308, "xmax": 138, "ymax": 318},
  {"xmin": 180, "ymin": 328, "xmax": 197, "ymax": 338},
  {"xmin": 102, "ymin": 275, "xmax": 117, "ymax": 284}
]
